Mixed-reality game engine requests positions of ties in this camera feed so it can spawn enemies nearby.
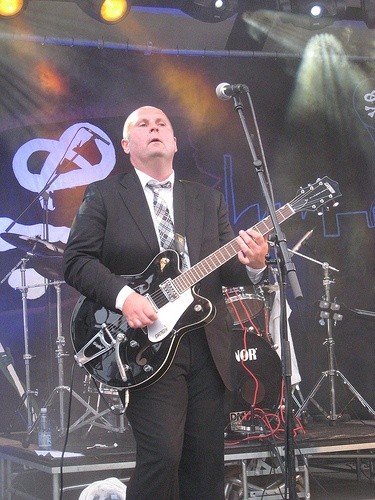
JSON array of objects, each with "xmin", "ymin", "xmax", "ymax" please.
[{"xmin": 145, "ymin": 180, "xmax": 188, "ymax": 273}]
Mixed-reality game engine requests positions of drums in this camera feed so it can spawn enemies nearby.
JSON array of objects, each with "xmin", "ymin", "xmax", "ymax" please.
[
  {"xmin": 80, "ymin": 372, "xmax": 120, "ymax": 395},
  {"xmin": 221, "ymin": 283, "xmax": 264, "ymax": 325},
  {"xmin": 227, "ymin": 329, "xmax": 284, "ymax": 434}
]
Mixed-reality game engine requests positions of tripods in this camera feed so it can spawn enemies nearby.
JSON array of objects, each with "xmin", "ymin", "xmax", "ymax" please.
[
  {"xmin": 1, "ymin": 138, "xmax": 120, "ymax": 449},
  {"xmin": 268, "ymin": 242, "xmax": 375, "ymax": 427}
]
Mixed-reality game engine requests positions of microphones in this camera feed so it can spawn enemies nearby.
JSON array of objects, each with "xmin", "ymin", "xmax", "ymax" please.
[
  {"xmin": 215, "ymin": 82, "xmax": 247, "ymax": 100},
  {"xmin": 84, "ymin": 127, "xmax": 110, "ymax": 145}
]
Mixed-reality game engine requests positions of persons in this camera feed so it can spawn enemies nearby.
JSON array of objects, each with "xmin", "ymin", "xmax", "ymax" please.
[{"xmin": 63, "ymin": 106, "xmax": 269, "ymax": 500}]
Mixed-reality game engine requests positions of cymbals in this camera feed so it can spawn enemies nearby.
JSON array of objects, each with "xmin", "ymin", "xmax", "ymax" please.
[
  {"xmin": 0, "ymin": 232, "xmax": 63, "ymax": 256},
  {"xmin": 35, "ymin": 257, "xmax": 66, "ymax": 280}
]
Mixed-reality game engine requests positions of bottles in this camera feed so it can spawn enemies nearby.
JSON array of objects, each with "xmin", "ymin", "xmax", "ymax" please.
[{"xmin": 37, "ymin": 408, "xmax": 52, "ymax": 451}]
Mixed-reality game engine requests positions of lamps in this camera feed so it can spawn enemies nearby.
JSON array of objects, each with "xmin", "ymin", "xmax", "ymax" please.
[
  {"xmin": 291, "ymin": 0, "xmax": 337, "ymax": 30},
  {"xmin": 177, "ymin": 0, "xmax": 239, "ymax": 23},
  {"xmin": 75, "ymin": 0, "xmax": 132, "ymax": 25},
  {"xmin": 0, "ymin": 0, "xmax": 27, "ymax": 19}
]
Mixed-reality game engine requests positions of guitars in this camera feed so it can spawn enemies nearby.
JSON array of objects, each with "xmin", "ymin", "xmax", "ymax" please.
[{"xmin": 70, "ymin": 173, "xmax": 345, "ymax": 393}]
